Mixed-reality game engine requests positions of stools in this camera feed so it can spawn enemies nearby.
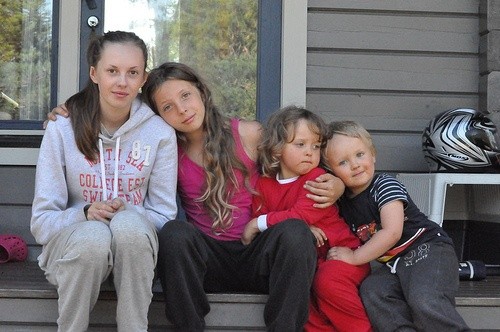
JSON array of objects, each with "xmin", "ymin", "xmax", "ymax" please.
[{"xmin": 396, "ymin": 173, "xmax": 500, "ymax": 228}]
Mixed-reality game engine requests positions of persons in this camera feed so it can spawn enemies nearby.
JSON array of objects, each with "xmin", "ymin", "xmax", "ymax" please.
[
  {"xmin": 29, "ymin": 31, "xmax": 178, "ymax": 332},
  {"xmin": 142, "ymin": 62, "xmax": 316, "ymax": 332},
  {"xmin": 240, "ymin": 104, "xmax": 372, "ymax": 332},
  {"xmin": 323, "ymin": 118, "xmax": 470, "ymax": 332}
]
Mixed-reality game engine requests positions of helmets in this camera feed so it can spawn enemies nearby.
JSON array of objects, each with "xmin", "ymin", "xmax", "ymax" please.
[{"xmin": 422, "ymin": 107, "xmax": 500, "ymax": 173}]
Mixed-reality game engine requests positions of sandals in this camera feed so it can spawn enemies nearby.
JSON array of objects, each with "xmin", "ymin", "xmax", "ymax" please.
[{"xmin": 0, "ymin": 235, "xmax": 28, "ymax": 263}]
